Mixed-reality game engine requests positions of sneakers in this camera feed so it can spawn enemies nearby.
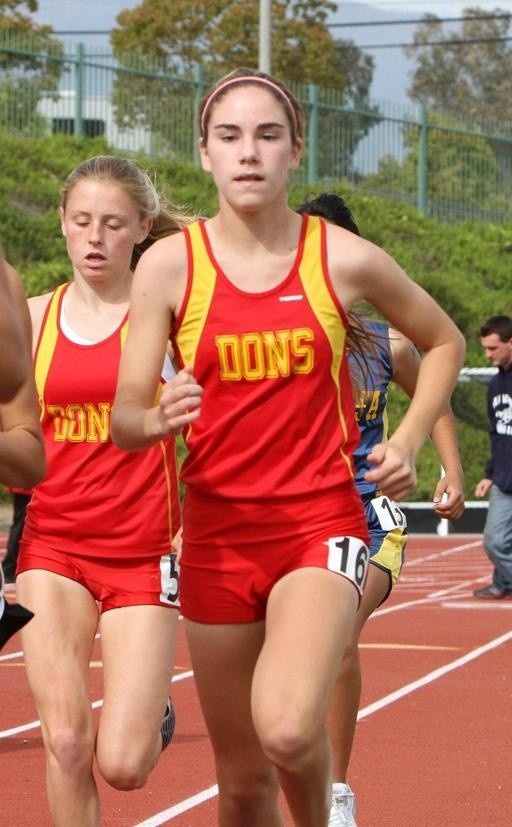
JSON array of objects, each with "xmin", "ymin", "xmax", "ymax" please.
[
  {"xmin": 473, "ymin": 583, "xmax": 512, "ymax": 600},
  {"xmin": 327, "ymin": 782, "xmax": 357, "ymax": 827}
]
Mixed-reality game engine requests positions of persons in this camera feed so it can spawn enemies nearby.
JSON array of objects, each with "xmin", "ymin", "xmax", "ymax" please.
[
  {"xmin": 107, "ymin": 67, "xmax": 467, "ymax": 824},
  {"xmin": 21, "ymin": 156, "xmax": 186, "ymax": 826},
  {"xmin": 0, "ymin": 251, "xmax": 49, "ymax": 493},
  {"xmin": 471, "ymin": 315, "xmax": 511, "ymax": 598},
  {"xmin": 228, "ymin": 195, "xmax": 465, "ymax": 825}
]
